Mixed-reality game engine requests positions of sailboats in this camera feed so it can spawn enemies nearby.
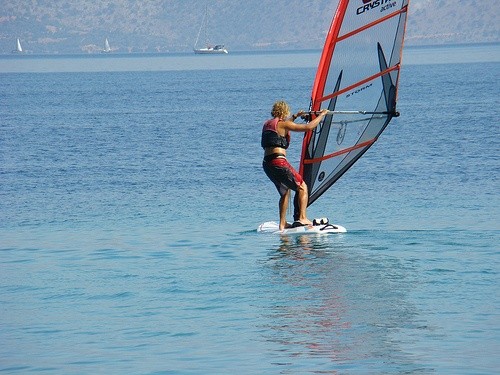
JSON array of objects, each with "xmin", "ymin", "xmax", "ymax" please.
[
  {"xmin": 256, "ymin": 0, "xmax": 410, "ymax": 236},
  {"xmin": 192, "ymin": 0, "xmax": 229, "ymax": 54},
  {"xmin": 15, "ymin": 37, "xmax": 23, "ymax": 54},
  {"xmin": 101, "ymin": 36, "xmax": 112, "ymax": 53}
]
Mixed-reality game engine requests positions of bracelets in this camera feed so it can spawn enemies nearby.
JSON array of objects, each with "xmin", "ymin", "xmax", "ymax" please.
[{"xmin": 292, "ymin": 115, "xmax": 296, "ymax": 119}]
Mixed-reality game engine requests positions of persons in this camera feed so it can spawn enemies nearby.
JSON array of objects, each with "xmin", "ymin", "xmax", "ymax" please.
[{"xmin": 261, "ymin": 100, "xmax": 329, "ymax": 230}]
